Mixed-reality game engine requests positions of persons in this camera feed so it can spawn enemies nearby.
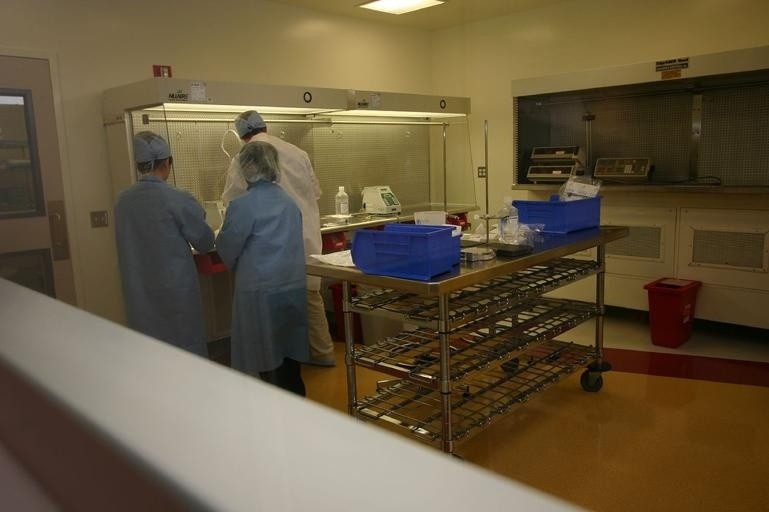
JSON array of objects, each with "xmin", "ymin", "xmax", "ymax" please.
[
  {"xmin": 113, "ymin": 131, "xmax": 215, "ymax": 359},
  {"xmin": 214, "ymin": 141, "xmax": 309, "ymax": 396},
  {"xmin": 221, "ymin": 110, "xmax": 336, "ymax": 367}
]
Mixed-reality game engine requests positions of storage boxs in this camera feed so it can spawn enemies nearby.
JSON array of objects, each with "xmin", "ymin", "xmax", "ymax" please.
[
  {"xmin": 350, "ymin": 223, "xmax": 463, "ymax": 280},
  {"xmin": 511, "ymin": 195, "xmax": 602, "ymax": 234}
]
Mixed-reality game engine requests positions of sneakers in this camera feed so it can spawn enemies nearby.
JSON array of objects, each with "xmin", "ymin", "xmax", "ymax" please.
[{"xmin": 303, "ymin": 353, "xmax": 337, "ymax": 368}]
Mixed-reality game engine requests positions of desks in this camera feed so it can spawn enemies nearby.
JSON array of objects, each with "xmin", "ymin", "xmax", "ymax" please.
[{"xmin": 305, "ymin": 226, "xmax": 631, "ymax": 452}]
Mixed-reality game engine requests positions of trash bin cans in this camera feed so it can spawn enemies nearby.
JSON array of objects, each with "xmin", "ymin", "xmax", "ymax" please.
[{"xmin": 642, "ymin": 275, "xmax": 701, "ymax": 350}]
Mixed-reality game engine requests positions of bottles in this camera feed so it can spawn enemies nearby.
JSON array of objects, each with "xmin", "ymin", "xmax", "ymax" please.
[
  {"xmin": 334, "ymin": 185, "xmax": 349, "ymax": 217},
  {"xmin": 497, "ymin": 196, "xmax": 520, "ymax": 242}
]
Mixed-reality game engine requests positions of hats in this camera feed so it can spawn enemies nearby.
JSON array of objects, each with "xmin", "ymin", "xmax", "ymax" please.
[
  {"xmin": 133, "ymin": 131, "xmax": 171, "ymax": 164},
  {"xmin": 235, "ymin": 110, "xmax": 267, "ymax": 139}
]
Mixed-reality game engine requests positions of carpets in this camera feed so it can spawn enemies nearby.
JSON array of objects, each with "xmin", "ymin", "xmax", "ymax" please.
[{"xmin": 280, "ymin": 337, "xmax": 769, "ymax": 512}]
